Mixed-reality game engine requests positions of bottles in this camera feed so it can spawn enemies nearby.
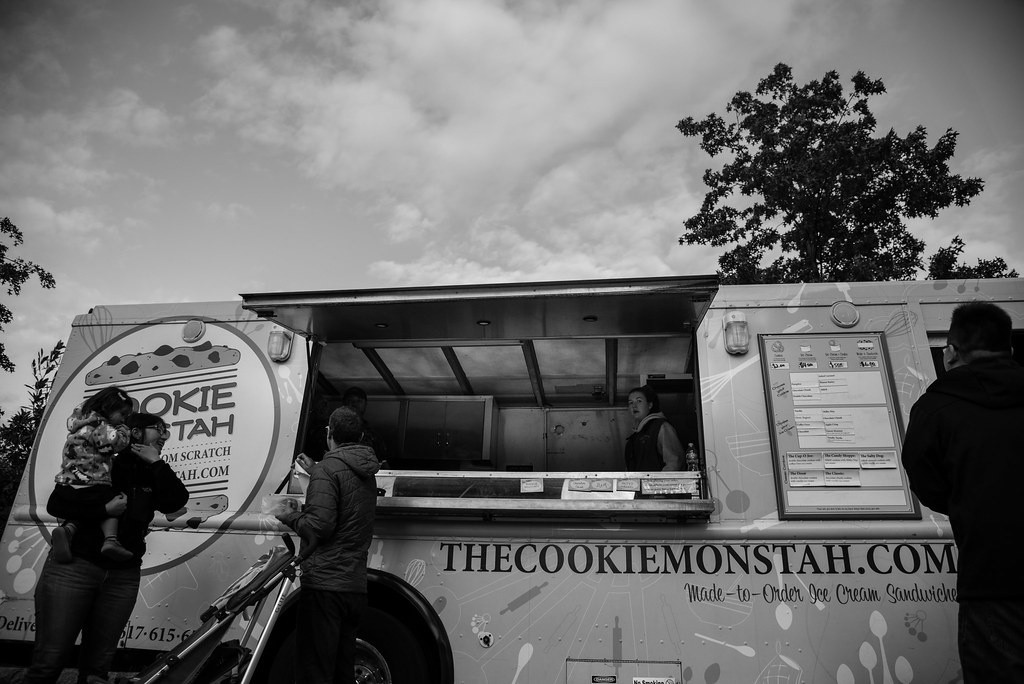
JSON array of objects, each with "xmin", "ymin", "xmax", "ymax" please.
[{"xmin": 685, "ymin": 443, "xmax": 699, "ymax": 471}]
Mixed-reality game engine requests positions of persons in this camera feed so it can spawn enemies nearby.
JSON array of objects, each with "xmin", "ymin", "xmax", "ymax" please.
[
  {"xmin": 22, "ymin": 386, "xmax": 189, "ymax": 684},
  {"xmin": 901, "ymin": 301, "xmax": 1024, "ymax": 684},
  {"xmin": 273, "ymin": 405, "xmax": 380, "ymax": 684},
  {"xmin": 624, "ymin": 387, "xmax": 684, "ymax": 473}
]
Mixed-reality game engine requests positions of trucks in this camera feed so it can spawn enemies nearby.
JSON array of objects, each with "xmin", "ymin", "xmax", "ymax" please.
[{"xmin": 0, "ymin": 274, "xmax": 1024, "ymax": 684}]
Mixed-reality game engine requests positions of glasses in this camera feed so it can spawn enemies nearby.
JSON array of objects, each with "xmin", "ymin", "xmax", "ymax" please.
[
  {"xmin": 939, "ymin": 344, "xmax": 958, "ymax": 354},
  {"xmin": 146, "ymin": 423, "xmax": 170, "ymax": 438}
]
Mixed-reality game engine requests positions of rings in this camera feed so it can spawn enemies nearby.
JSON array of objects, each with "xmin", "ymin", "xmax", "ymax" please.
[{"xmin": 138, "ymin": 448, "xmax": 141, "ymax": 452}]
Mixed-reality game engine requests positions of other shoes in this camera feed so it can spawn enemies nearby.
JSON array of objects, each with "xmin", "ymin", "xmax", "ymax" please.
[
  {"xmin": 52, "ymin": 526, "xmax": 73, "ymax": 564},
  {"xmin": 101, "ymin": 541, "xmax": 133, "ymax": 561}
]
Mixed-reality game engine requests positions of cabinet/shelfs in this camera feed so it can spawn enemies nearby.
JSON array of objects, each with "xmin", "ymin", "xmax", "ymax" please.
[{"xmin": 322, "ymin": 395, "xmax": 499, "ymax": 466}]
